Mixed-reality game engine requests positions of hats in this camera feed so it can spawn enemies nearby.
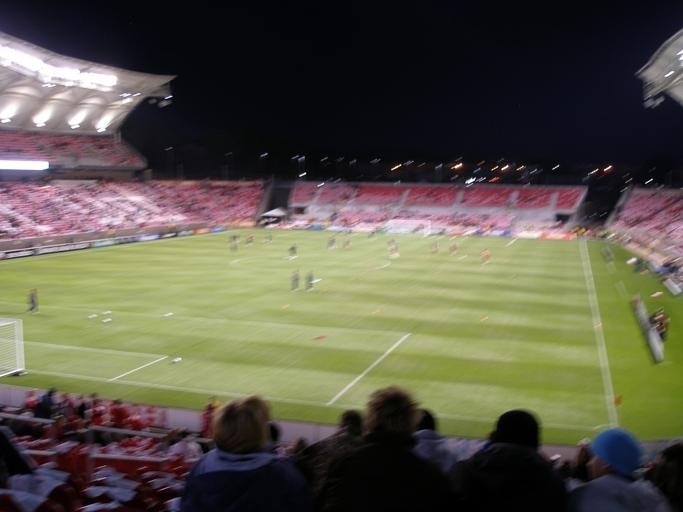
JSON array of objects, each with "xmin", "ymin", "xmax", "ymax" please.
[
  {"xmin": 590, "ymin": 425, "xmax": 647, "ymax": 477},
  {"xmin": 491, "ymin": 404, "xmax": 545, "ymax": 453}
]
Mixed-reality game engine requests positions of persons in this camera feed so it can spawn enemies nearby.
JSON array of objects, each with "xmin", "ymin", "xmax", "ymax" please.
[
  {"xmin": 650, "ymin": 304, "xmax": 671, "ymax": 339},
  {"xmin": 1, "ymin": 384, "xmax": 682, "ymax": 512},
  {"xmin": 1, "ymin": 127, "xmax": 682, "ymax": 314}
]
